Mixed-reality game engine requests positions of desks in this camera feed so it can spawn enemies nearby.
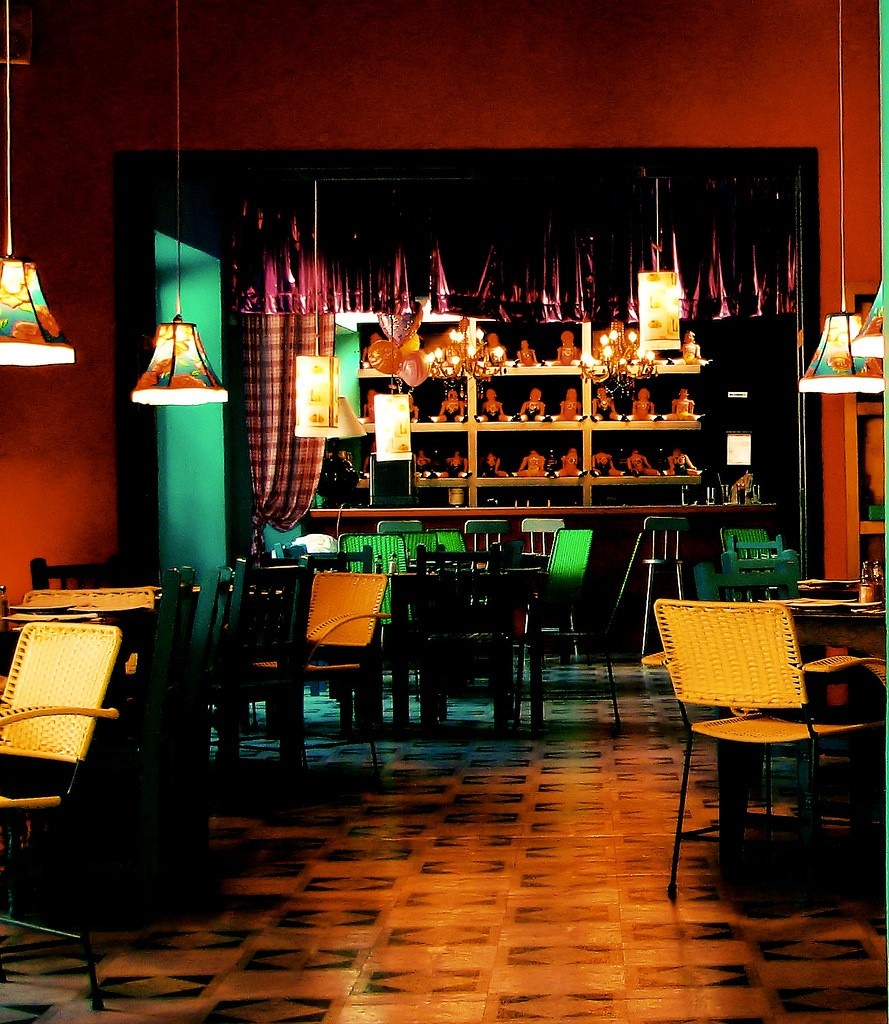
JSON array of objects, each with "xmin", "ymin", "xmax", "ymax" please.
[
  {"xmin": 375, "ymin": 569, "xmax": 544, "ymax": 734},
  {"xmin": 716, "ymin": 597, "xmax": 885, "ymax": 897}
]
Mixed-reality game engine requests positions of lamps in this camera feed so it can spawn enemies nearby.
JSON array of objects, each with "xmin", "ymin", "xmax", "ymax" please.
[
  {"xmin": 296, "ymin": 181, "xmax": 341, "ymax": 438},
  {"xmin": 0, "ymin": 0, "xmax": 79, "ymax": 370},
  {"xmin": 638, "ymin": 178, "xmax": 683, "ymax": 352},
  {"xmin": 797, "ymin": 0, "xmax": 885, "ymax": 393},
  {"xmin": 374, "ymin": 178, "xmax": 415, "ymax": 463},
  {"xmin": 130, "ymin": 0, "xmax": 230, "ymax": 404}
]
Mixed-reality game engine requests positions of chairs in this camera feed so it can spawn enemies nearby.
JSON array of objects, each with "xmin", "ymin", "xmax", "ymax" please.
[{"xmin": 1, "ymin": 516, "xmax": 886, "ymax": 1014}]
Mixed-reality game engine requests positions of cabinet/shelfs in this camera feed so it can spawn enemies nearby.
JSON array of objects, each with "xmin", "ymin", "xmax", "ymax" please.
[
  {"xmin": 357, "ymin": 319, "xmax": 703, "ymax": 507},
  {"xmin": 841, "ymin": 276, "xmax": 884, "ymax": 579}
]
